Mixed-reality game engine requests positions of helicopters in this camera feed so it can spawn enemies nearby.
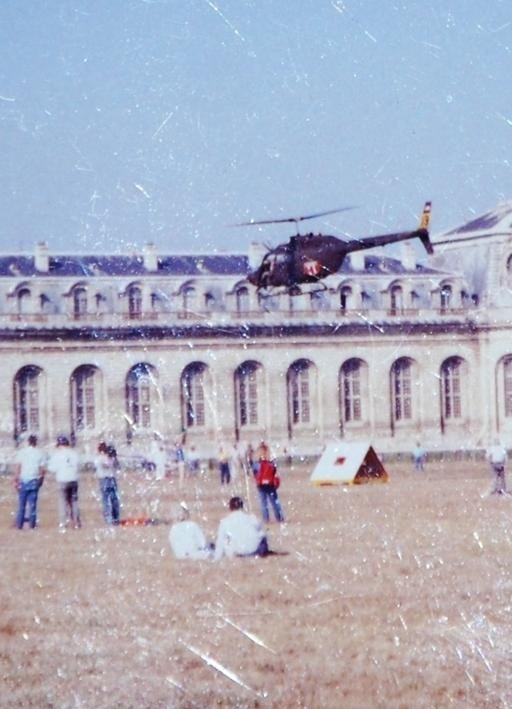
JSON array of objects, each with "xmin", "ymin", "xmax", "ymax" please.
[{"xmin": 229, "ymin": 201, "xmax": 434, "ymax": 299}]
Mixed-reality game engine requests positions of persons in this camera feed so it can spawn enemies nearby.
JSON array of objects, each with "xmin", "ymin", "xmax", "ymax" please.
[
  {"xmin": 411, "ymin": 439, "xmax": 431, "ymax": 473},
  {"xmin": 14, "ymin": 420, "xmax": 295, "ymax": 562},
  {"xmin": 484, "ymin": 438, "xmax": 508, "ymax": 497}
]
